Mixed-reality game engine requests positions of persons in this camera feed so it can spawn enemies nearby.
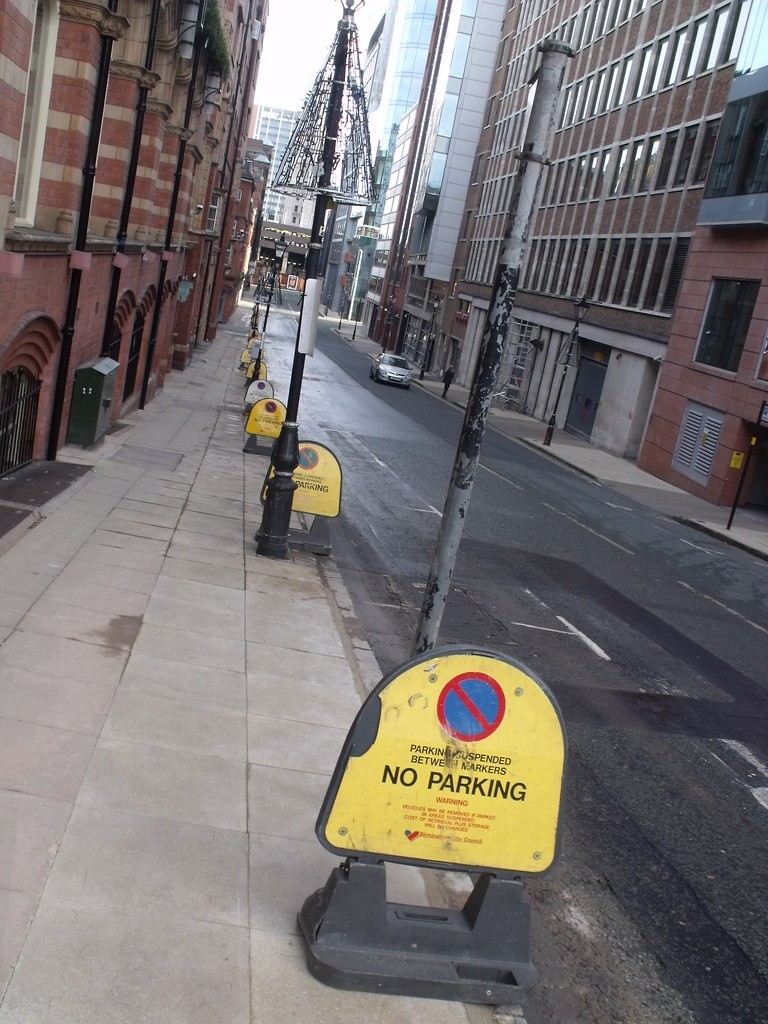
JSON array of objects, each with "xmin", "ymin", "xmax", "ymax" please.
[{"xmin": 441, "ymin": 364, "xmax": 455, "ymax": 399}]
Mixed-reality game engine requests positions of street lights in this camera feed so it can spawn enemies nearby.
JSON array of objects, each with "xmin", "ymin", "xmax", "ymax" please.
[
  {"xmin": 540, "ymin": 294, "xmax": 590, "ymax": 446},
  {"xmin": 254, "ymin": 0, "xmax": 371, "ymax": 559},
  {"xmin": 382, "ymin": 295, "xmax": 397, "ymax": 353},
  {"xmin": 418, "ymin": 293, "xmax": 441, "ymax": 381},
  {"xmin": 252, "ymin": 233, "xmax": 289, "ymax": 382}
]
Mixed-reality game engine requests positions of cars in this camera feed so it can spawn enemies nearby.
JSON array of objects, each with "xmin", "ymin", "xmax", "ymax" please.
[{"xmin": 368, "ymin": 352, "xmax": 415, "ymax": 389}]
